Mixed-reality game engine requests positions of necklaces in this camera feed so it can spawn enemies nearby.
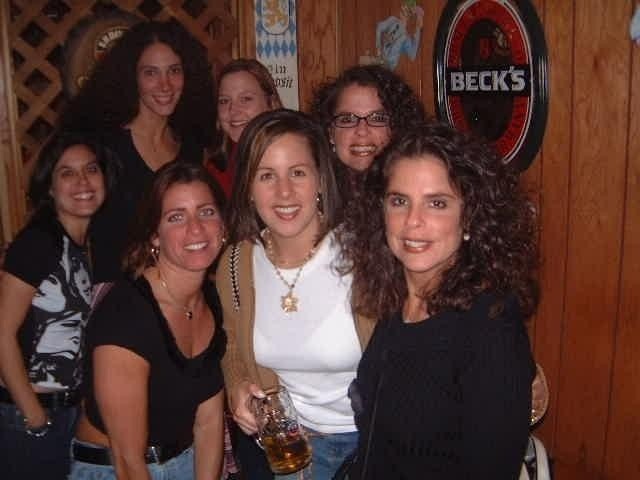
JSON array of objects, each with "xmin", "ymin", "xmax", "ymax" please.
[
  {"xmin": 158, "ymin": 266, "xmax": 202, "ymax": 320},
  {"xmin": 265, "ymin": 210, "xmax": 323, "ymax": 313}
]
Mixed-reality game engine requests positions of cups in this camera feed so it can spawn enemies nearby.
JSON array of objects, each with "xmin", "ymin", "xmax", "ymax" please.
[{"xmin": 244, "ymin": 386, "xmax": 313, "ymax": 475}]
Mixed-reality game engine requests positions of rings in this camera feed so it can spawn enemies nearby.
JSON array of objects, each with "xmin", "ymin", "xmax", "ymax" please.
[{"xmin": 530, "ymin": 415, "xmax": 538, "ymax": 424}]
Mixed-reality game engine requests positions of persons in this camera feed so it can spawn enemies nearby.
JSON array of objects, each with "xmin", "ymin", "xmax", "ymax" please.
[
  {"xmin": 328, "ymin": 119, "xmax": 540, "ymax": 478},
  {"xmin": 310, "ymin": 66, "xmax": 428, "ymax": 189},
  {"xmin": 58, "ymin": 18, "xmax": 213, "ymax": 269},
  {"xmin": 0, "ymin": 131, "xmax": 128, "ymax": 479},
  {"xmin": 201, "ymin": 56, "xmax": 283, "ymax": 201},
  {"xmin": 65, "ymin": 159, "xmax": 229, "ymax": 480},
  {"xmin": 213, "ymin": 107, "xmax": 548, "ymax": 479}
]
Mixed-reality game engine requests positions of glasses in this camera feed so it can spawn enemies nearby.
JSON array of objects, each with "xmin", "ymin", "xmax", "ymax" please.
[{"xmin": 328, "ymin": 112, "xmax": 393, "ymax": 128}]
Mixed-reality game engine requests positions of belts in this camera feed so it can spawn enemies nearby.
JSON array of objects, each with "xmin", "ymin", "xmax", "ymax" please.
[
  {"xmin": 72, "ymin": 444, "xmax": 184, "ymax": 465},
  {"xmin": 0, "ymin": 388, "xmax": 73, "ymax": 407}
]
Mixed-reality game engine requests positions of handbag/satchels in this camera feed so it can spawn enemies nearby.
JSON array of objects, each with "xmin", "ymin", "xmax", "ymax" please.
[{"xmin": 519, "ymin": 434, "xmax": 549, "ymax": 480}]
